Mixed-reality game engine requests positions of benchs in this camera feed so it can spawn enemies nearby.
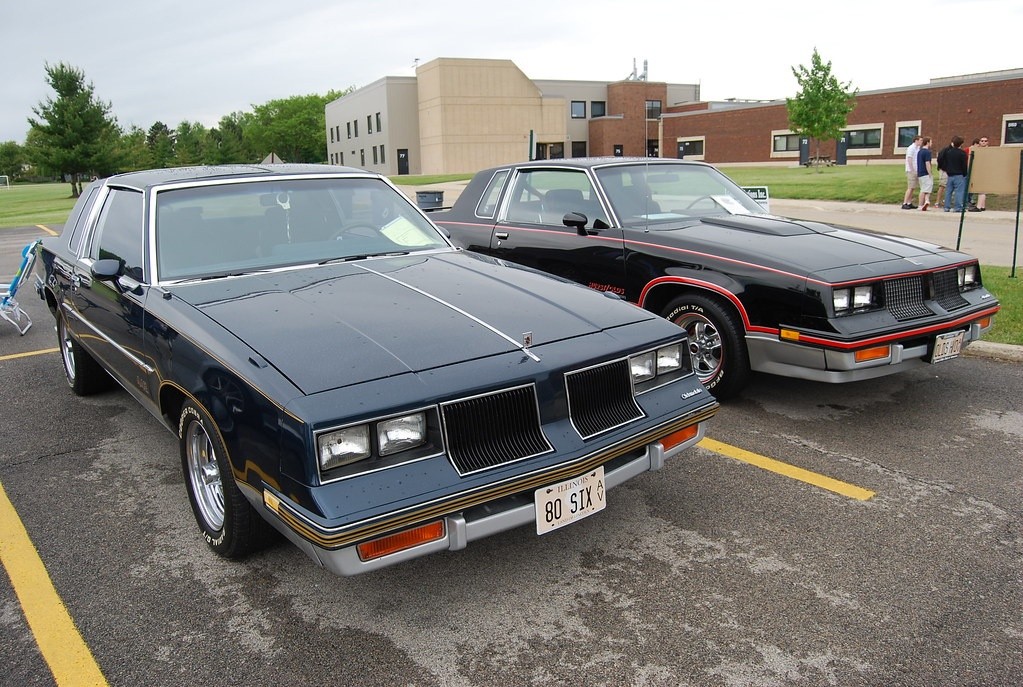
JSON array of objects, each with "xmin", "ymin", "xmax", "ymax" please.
[
  {"xmin": 802, "ymin": 162, "xmax": 812, "ymax": 165},
  {"xmin": 825, "ymin": 161, "xmax": 836, "ymax": 165}
]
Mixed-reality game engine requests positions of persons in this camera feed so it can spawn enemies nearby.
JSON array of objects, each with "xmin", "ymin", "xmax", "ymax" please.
[
  {"xmin": 917, "ymin": 136, "xmax": 933, "ymax": 211},
  {"xmin": 901, "ymin": 136, "xmax": 923, "ymax": 209},
  {"xmin": 934, "ymin": 135, "xmax": 988, "ymax": 212}
]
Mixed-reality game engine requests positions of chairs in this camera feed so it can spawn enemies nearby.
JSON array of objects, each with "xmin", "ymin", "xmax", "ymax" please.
[
  {"xmin": 0, "ymin": 237, "xmax": 43, "ymax": 337},
  {"xmin": 544, "ymin": 180, "xmax": 660, "ymax": 221},
  {"xmin": 159, "ymin": 192, "xmax": 345, "ymax": 274}
]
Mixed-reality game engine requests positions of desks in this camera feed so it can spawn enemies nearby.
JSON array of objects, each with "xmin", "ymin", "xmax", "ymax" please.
[{"xmin": 808, "ymin": 156, "xmax": 832, "ymax": 169}]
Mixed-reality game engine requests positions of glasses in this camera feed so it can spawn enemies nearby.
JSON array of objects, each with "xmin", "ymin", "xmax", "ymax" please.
[{"xmin": 981, "ymin": 140, "xmax": 987, "ymax": 142}]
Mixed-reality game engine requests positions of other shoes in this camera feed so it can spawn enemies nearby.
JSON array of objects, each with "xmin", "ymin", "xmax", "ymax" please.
[
  {"xmin": 968, "ymin": 207, "xmax": 985, "ymax": 212},
  {"xmin": 956, "ymin": 209, "xmax": 966, "ymax": 212},
  {"xmin": 933, "ymin": 203, "xmax": 939, "ymax": 208},
  {"xmin": 902, "ymin": 203, "xmax": 918, "ymax": 209},
  {"xmin": 918, "ymin": 205, "xmax": 923, "ymax": 210},
  {"xmin": 922, "ymin": 201, "xmax": 930, "ymax": 211}
]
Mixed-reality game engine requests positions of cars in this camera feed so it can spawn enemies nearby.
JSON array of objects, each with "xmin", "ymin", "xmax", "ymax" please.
[
  {"xmin": 422, "ymin": 156, "xmax": 1001, "ymax": 403},
  {"xmin": 34, "ymin": 163, "xmax": 721, "ymax": 577}
]
